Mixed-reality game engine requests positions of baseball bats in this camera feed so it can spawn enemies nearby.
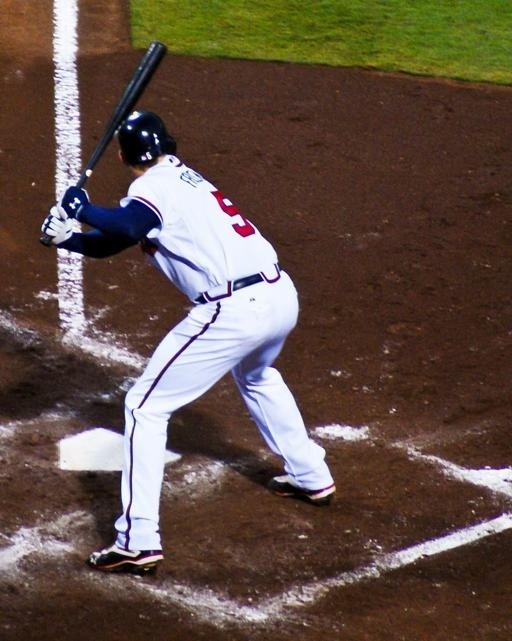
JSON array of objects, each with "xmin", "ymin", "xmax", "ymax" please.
[{"xmin": 39, "ymin": 39, "xmax": 166, "ymax": 246}]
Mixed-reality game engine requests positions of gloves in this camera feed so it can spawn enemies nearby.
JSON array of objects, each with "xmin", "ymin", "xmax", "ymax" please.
[{"xmin": 41, "ymin": 188, "xmax": 90, "ymax": 249}]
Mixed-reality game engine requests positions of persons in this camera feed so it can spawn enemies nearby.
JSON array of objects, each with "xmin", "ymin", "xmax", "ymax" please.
[{"xmin": 36, "ymin": 106, "xmax": 338, "ymax": 574}]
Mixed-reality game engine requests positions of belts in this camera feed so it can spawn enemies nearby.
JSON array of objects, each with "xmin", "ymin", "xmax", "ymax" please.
[{"xmin": 198, "ymin": 262, "xmax": 281, "ymax": 306}]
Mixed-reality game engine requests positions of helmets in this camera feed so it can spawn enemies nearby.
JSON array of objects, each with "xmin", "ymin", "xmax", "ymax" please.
[{"xmin": 116, "ymin": 109, "xmax": 176, "ymax": 165}]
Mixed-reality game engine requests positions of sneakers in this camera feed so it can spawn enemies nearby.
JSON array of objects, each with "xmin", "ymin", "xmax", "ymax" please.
[
  {"xmin": 267, "ymin": 474, "xmax": 337, "ymax": 504},
  {"xmin": 85, "ymin": 541, "xmax": 165, "ymax": 572}
]
